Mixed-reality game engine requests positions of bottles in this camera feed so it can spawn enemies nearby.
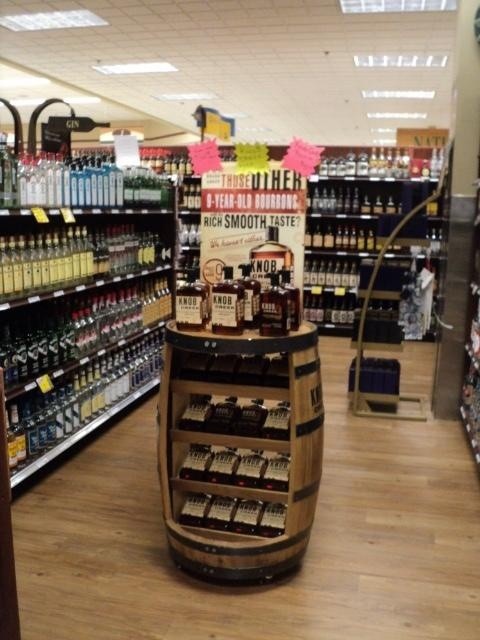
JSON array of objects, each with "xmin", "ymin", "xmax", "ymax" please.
[
  {"xmin": 0, "ymin": 133, "xmax": 178, "ymax": 208},
  {"xmin": 1, "ymin": 227, "xmax": 171, "ymax": 303},
  {"xmin": 207, "ymin": 447, "xmax": 241, "ymax": 484},
  {"xmin": 304, "ymin": 290, "xmax": 399, "ymax": 330},
  {"xmin": 427, "ymin": 191, "xmax": 438, "ymax": 216},
  {"xmin": 182, "ymin": 185, "xmax": 188, "ymax": 209},
  {"xmin": 177, "ymin": 394, "xmax": 215, "ymax": 431},
  {"xmin": 262, "ymin": 401, "xmax": 290, "ymax": 440},
  {"xmin": 189, "ymin": 225, "xmax": 197, "ymax": 246},
  {"xmin": 186, "ymin": 159, "xmax": 193, "ymax": 174},
  {"xmin": 464, "ymin": 367, "xmax": 477, "ymax": 408},
  {"xmin": 304, "ymin": 224, "xmax": 446, "ymax": 255},
  {"xmin": 304, "ymin": 256, "xmax": 361, "ymax": 288},
  {"xmin": 180, "ymin": 223, "xmax": 201, "ymax": 245},
  {"xmin": 307, "ymin": 186, "xmax": 403, "ymax": 215},
  {"xmin": 179, "ymin": 492, "xmax": 215, "ymax": 527},
  {"xmin": 177, "ymin": 254, "xmax": 200, "ymax": 282},
  {"xmin": 196, "ymin": 226, "xmax": 201, "ymax": 245},
  {"xmin": 192, "ymin": 266, "xmax": 210, "ymax": 320},
  {"xmin": 205, "ymin": 496, "xmax": 239, "ymax": 531},
  {"xmin": 188, "ymin": 185, "xmax": 195, "ymax": 210},
  {"xmin": 278, "ymin": 270, "xmax": 302, "ymax": 331},
  {"xmin": 206, "ymin": 396, "xmax": 241, "ymax": 433},
  {"xmin": 181, "ymin": 225, "xmax": 188, "ymax": 246},
  {"xmin": 263, "ymin": 452, "xmax": 289, "ymax": 492},
  {"xmin": 234, "ymin": 264, "xmax": 262, "ymax": 330},
  {"xmin": 0, "ymin": 267, "xmax": 172, "ymax": 390},
  {"xmin": 234, "ymin": 399, "xmax": 268, "ymax": 436},
  {"xmin": 443, "ymin": 189, "xmax": 450, "ymax": 216},
  {"xmin": 234, "ymin": 449, "xmax": 269, "ymax": 487},
  {"xmin": 250, "ymin": 226, "xmax": 295, "ymax": 289},
  {"xmin": 5, "ymin": 327, "xmax": 167, "ymax": 477},
  {"xmin": 179, "ymin": 444, "xmax": 214, "ymax": 481},
  {"xmin": 321, "ymin": 147, "xmax": 445, "ymax": 178},
  {"xmin": 348, "ymin": 356, "xmax": 400, "ymax": 412},
  {"xmin": 233, "ymin": 500, "xmax": 266, "ymax": 535},
  {"xmin": 174, "ymin": 269, "xmax": 207, "ymax": 332},
  {"xmin": 259, "ymin": 272, "xmax": 291, "ymax": 336},
  {"xmin": 178, "ymin": 151, "xmax": 186, "ymax": 174},
  {"xmin": 212, "ymin": 267, "xmax": 245, "ymax": 336},
  {"xmin": 195, "ymin": 184, "xmax": 202, "ymax": 210}
]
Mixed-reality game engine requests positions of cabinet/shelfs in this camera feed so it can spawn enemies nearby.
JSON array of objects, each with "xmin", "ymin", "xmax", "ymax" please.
[
  {"xmin": 0, "ymin": 185, "xmax": 179, "ymax": 489},
  {"xmin": 157, "ymin": 319, "xmax": 325, "ymax": 582},
  {"xmin": 70, "ymin": 143, "xmax": 455, "ymax": 335}
]
